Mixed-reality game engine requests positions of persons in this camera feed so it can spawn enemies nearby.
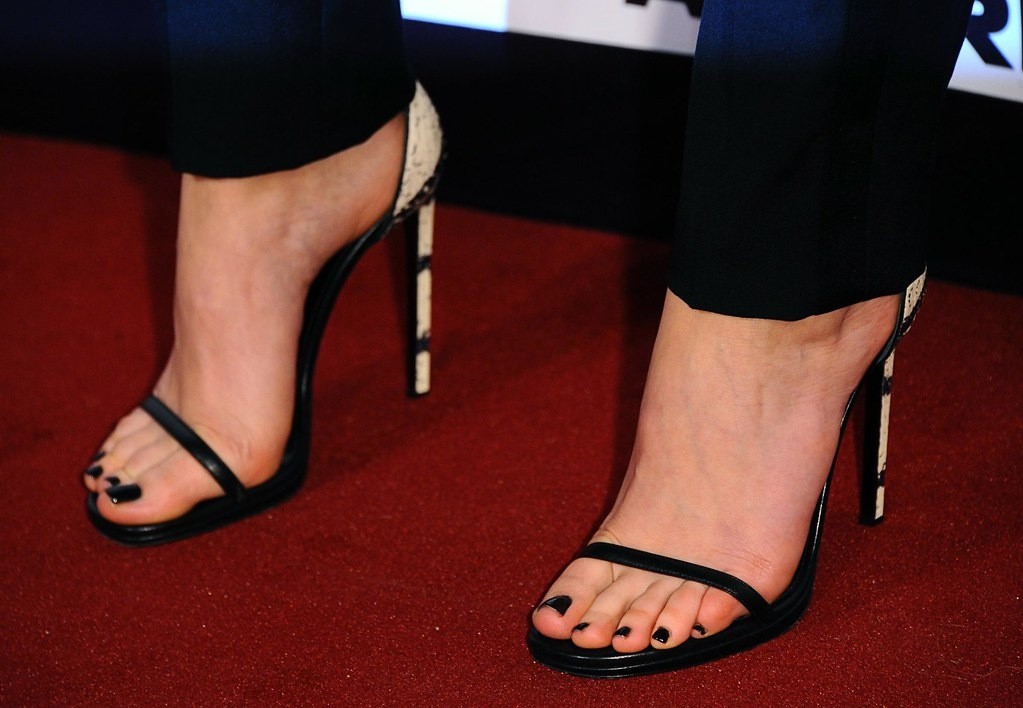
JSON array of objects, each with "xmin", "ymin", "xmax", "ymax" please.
[{"xmin": 0, "ymin": 0, "xmax": 975, "ymax": 679}]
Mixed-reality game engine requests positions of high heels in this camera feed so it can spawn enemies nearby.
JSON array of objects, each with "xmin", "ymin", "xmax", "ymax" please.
[
  {"xmin": 86, "ymin": 81, "xmax": 444, "ymax": 547},
  {"xmin": 527, "ymin": 266, "xmax": 927, "ymax": 678}
]
